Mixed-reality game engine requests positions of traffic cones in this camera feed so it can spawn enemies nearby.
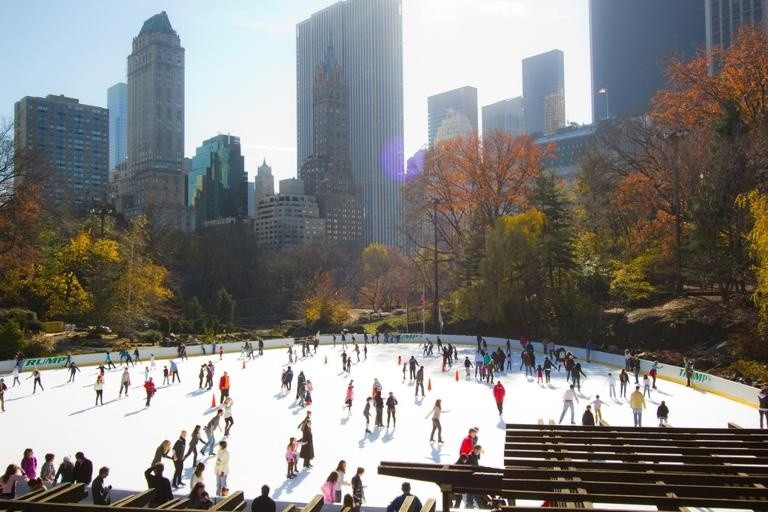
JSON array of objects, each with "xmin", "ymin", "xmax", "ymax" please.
[
  {"xmin": 242, "ymin": 360, "xmax": 246, "ymax": 368},
  {"xmin": 324, "ymin": 355, "xmax": 328, "ymax": 364},
  {"xmin": 428, "ymin": 377, "xmax": 431, "ymax": 391},
  {"xmin": 455, "ymin": 370, "xmax": 459, "ymax": 382},
  {"xmin": 211, "ymin": 392, "xmax": 216, "ymax": 408}
]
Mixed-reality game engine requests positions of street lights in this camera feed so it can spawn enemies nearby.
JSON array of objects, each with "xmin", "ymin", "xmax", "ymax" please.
[
  {"xmin": 432, "ymin": 196, "xmax": 442, "ymax": 335},
  {"xmin": 89, "ymin": 203, "xmax": 112, "ymax": 336},
  {"xmin": 661, "ymin": 127, "xmax": 693, "ymax": 295}
]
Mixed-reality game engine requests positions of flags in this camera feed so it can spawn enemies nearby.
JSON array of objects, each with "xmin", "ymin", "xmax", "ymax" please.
[
  {"xmin": 438, "ymin": 307, "xmax": 444, "ymax": 328},
  {"xmin": 419, "ymin": 289, "xmax": 425, "ymax": 305}
]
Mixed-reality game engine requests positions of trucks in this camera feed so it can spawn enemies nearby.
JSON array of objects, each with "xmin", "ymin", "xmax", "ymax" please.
[{"xmin": 88, "ymin": 324, "xmax": 112, "ymax": 335}]
